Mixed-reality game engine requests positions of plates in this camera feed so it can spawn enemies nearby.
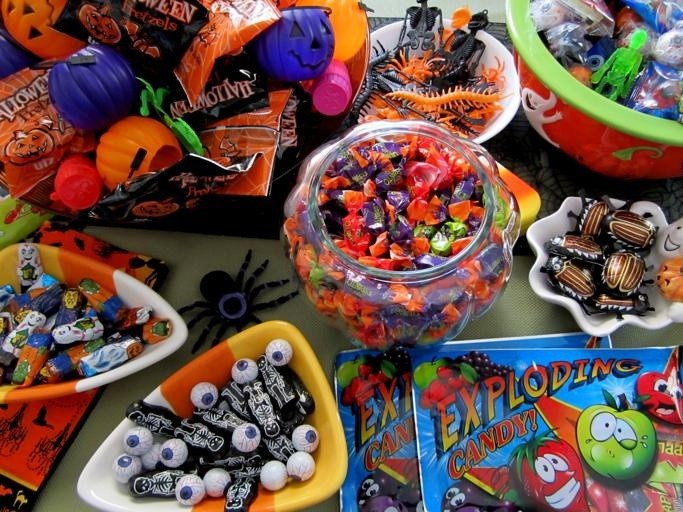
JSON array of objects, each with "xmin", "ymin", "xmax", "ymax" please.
[
  {"xmin": 525, "ymin": 194, "xmax": 683, "ymax": 339},
  {"xmin": 77, "ymin": 318, "xmax": 348, "ymax": 511},
  {"xmin": 1, "ymin": 242, "xmax": 188, "ymax": 406},
  {"xmin": 354, "ymin": 17, "xmax": 522, "ymax": 147}
]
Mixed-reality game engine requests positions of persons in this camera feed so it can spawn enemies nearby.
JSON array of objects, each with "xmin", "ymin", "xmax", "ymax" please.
[
  {"xmin": 591, "ymin": 29, "xmax": 647, "ymax": 101},
  {"xmin": 396, "ymin": 2, "xmax": 488, "ymax": 77}
]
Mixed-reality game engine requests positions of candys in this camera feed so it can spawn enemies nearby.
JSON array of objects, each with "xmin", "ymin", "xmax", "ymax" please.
[{"xmin": 0, "ymin": 132, "xmax": 662, "ymax": 512}]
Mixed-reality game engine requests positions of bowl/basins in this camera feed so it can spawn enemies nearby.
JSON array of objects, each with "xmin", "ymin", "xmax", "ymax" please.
[{"xmin": 499, "ymin": 1, "xmax": 683, "ymax": 182}]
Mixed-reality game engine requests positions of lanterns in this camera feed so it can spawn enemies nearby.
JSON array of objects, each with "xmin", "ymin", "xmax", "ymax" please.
[
  {"xmin": 251, "ymin": 2, "xmax": 368, "ymax": 83},
  {"xmin": 1, "ymin": 1, "xmax": 183, "ymax": 193}
]
